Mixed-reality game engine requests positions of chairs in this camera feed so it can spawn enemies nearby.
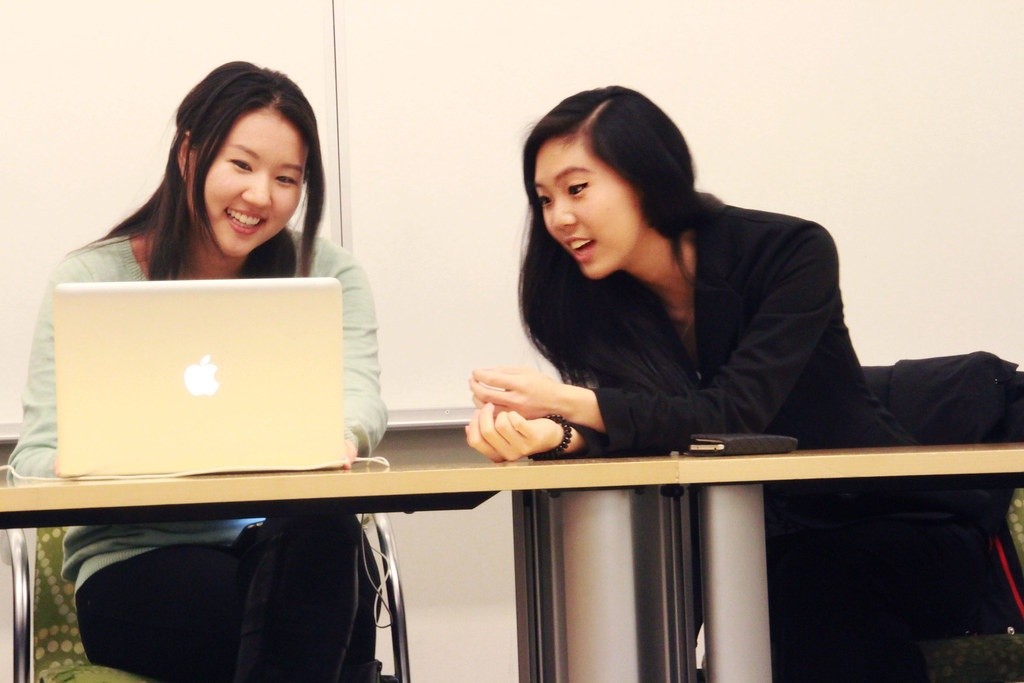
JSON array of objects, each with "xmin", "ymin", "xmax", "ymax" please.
[
  {"xmin": 861, "ymin": 350, "xmax": 1024, "ymax": 683},
  {"xmin": 6, "ymin": 512, "xmax": 411, "ymax": 683}
]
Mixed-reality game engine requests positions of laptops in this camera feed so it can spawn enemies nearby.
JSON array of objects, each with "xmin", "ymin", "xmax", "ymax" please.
[{"xmin": 53, "ymin": 275, "xmax": 345, "ymax": 480}]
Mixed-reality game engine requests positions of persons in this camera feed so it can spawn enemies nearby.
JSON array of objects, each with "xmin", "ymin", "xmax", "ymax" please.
[
  {"xmin": 466, "ymin": 85, "xmax": 987, "ymax": 683},
  {"xmin": 7, "ymin": 63, "xmax": 389, "ymax": 683}
]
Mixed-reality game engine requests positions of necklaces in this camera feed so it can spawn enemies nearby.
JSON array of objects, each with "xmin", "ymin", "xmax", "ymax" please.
[{"xmin": 681, "ymin": 311, "xmax": 695, "ymax": 338}]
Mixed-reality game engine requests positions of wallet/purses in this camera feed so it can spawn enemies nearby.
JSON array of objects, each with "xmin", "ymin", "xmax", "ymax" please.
[{"xmin": 689, "ymin": 434, "xmax": 798, "ymax": 457}]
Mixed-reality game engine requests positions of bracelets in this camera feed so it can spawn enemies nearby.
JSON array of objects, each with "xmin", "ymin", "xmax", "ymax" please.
[{"xmin": 529, "ymin": 413, "xmax": 571, "ymax": 458}]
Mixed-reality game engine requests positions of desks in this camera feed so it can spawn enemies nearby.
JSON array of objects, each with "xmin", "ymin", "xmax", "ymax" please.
[{"xmin": 0, "ymin": 442, "xmax": 1024, "ymax": 683}]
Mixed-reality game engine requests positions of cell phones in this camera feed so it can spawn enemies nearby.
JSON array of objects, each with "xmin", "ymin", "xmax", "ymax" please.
[{"xmin": 689, "ymin": 443, "xmax": 723, "ymax": 452}]
